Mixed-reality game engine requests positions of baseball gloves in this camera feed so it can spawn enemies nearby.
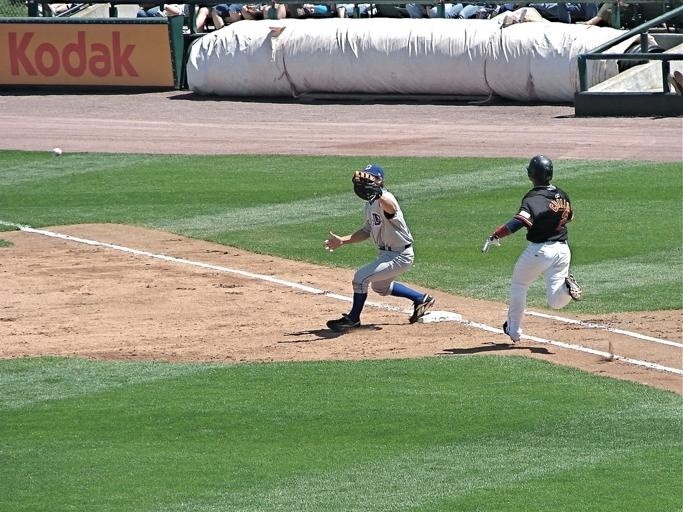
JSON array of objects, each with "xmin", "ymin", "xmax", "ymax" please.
[{"xmin": 352, "ymin": 171, "xmax": 382, "ymax": 205}]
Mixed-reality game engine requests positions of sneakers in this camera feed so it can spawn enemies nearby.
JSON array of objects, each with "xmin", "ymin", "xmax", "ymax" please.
[
  {"xmin": 408, "ymin": 294, "xmax": 435, "ymax": 323},
  {"xmin": 565, "ymin": 273, "xmax": 582, "ymax": 301},
  {"xmin": 327, "ymin": 313, "xmax": 360, "ymax": 331},
  {"xmin": 670, "ymin": 71, "xmax": 683, "ymax": 96},
  {"xmin": 503, "ymin": 320, "xmax": 521, "ymax": 343}
]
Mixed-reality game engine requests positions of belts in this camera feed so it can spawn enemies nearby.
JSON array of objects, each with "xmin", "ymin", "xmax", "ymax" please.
[{"xmin": 379, "ymin": 244, "xmax": 411, "ymax": 251}]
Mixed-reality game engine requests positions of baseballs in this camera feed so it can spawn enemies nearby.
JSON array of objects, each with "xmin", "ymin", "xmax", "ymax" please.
[{"xmin": 52, "ymin": 147, "xmax": 63, "ymax": 157}]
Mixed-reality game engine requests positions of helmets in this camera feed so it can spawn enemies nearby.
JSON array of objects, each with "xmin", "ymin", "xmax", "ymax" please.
[{"xmin": 527, "ymin": 155, "xmax": 554, "ymax": 182}]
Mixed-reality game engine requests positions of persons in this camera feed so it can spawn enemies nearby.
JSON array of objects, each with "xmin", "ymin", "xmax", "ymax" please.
[
  {"xmin": 481, "ymin": 155, "xmax": 584, "ymax": 342},
  {"xmin": 323, "ymin": 164, "xmax": 435, "ymax": 330},
  {"xmin": 136, "ymin": 1, "xmax": 682, "ymax": 32}
]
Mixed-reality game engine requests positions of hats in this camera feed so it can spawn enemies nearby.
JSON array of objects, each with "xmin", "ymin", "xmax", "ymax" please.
[{"xmin": 364, "ymin": 164, "xmax": 384, "ymax": 178}]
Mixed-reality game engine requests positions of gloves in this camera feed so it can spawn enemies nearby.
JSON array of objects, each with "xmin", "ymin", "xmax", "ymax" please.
[{"xmin": 482, "ymin": 233, "xmax": 501, "ymax": 254}]
